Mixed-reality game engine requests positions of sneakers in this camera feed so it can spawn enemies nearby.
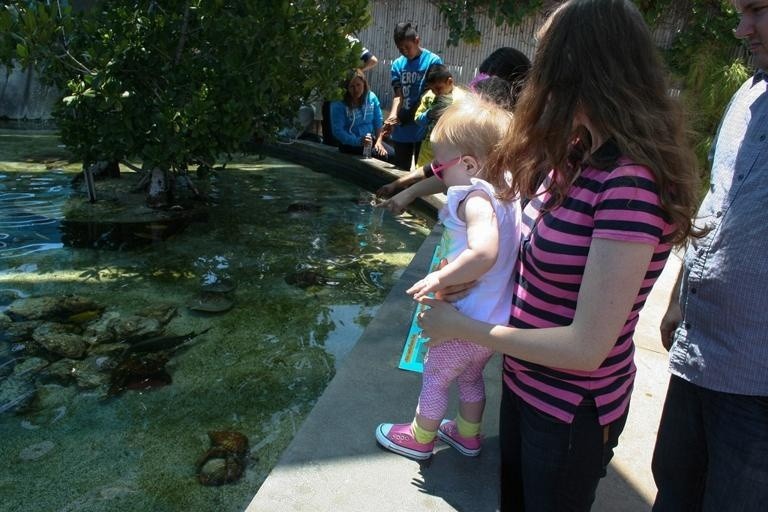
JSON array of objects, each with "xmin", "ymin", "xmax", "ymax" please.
[
  {"xmin": 375, "ymin": 422, "xmax": 438, "ymax": 460},
  {"xmin": 437, "ymin": 418, "xmax": 482, "ymax": 457}
]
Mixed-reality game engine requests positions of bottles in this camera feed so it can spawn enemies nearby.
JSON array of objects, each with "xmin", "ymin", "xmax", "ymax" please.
[{"xmin": 363, "ymin": 133, "xmax": 373, "ymax": 159}]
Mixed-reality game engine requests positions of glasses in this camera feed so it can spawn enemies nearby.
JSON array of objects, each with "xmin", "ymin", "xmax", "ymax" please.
[{"xmin": 430, "ymin": 157, "xmax": 463, "ymax": 181}]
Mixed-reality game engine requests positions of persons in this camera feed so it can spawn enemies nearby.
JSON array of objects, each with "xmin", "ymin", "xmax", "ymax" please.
[
  {"xmin": 650, "ymin": 0, "xmax": 768, "ymax": 511},
  {"xmin": 371, "ymin": 90, "xmax": 516, "ymax": 464},
  {"xmin": 277, "ymin": 19, "xmax": 532, "ymax": 216},
  {"xmin": 415, "ymin": 0, "xmax": 714, "ymax": 512}
]
